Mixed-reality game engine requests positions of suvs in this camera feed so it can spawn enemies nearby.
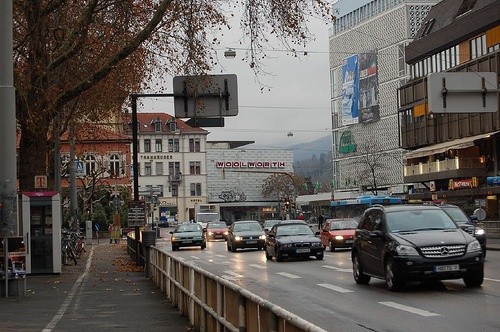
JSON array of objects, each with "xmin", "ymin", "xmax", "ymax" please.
[{"xmin": 351, "ymin": 204, "xmax": 484, "ymax": 292}]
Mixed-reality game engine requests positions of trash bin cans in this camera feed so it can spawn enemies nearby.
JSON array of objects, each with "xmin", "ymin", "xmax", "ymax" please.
[{"xmin": 155, "ymin": 228, "xmax": 160, "ymax": 237}]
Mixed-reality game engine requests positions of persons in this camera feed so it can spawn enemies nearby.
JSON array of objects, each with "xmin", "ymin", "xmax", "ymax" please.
[
  {"xmin": 109, "ymin": 224, "xmax": 118, "ymax": 244},
  {"xmin": 319, "ymin": 215, "xmax": 324, "ymax": 230}
]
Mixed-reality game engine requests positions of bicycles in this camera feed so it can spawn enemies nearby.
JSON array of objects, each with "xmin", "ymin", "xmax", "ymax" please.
[{"xmin": 61, "ymin": 227, "xmax": 86, "ymax": 267}]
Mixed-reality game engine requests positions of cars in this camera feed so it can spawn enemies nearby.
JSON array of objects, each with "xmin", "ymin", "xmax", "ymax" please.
[
  {"xmin": 205, "ymin": 221, "xmax": 231, "ymax": 242},
  {"xmin": 265, "ymin": 222, "xmax": 325, "ymax": 262},
  {"xmin": 169, "ymin": 223, "xmax": 207, "ymax": 251},
  {"xmin": 320, "ymin": 217, "xmax": 358, "ymax": 252},
  {"xmin": 437, "ymin": 203, "xmax": 487, "ymax": 259},
  {"xmin": 262, "ymin": 219, "xmax": 322, "ymax": 238},
  {"xmin": 227, "ymin": 220, "xmax": 267, "ymax": 252}
]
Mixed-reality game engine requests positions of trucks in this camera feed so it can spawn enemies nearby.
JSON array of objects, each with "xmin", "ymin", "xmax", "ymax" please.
[{"xmin": 194, "ymin": 203, "xmax": 221, "ymax": 232}]
